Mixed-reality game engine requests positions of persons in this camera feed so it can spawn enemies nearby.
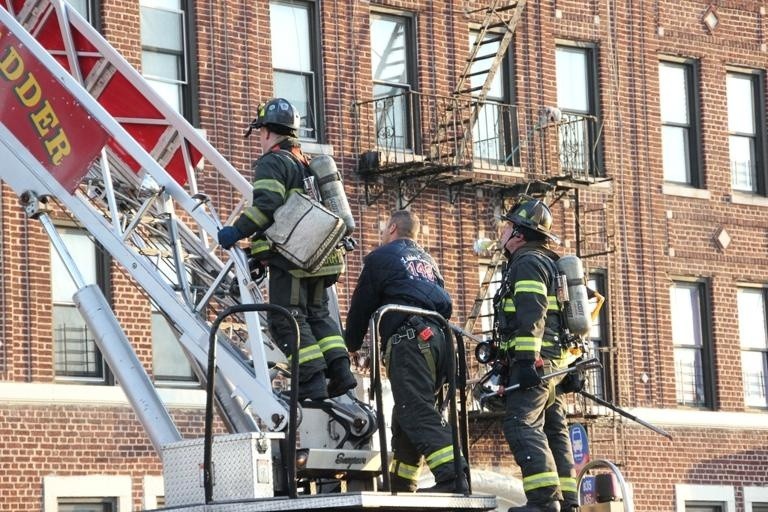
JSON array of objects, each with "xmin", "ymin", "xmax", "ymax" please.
[
  {"xmin": 492, "ymin": 202, "xmax": 588, "ymax": 511},
  {"xmin": 219, "ymin": 98, "xmax": 358, "ymax": 400},
  {"xmin": 345, "ymin": 211, "xmax": 469, "ymax": 493}
]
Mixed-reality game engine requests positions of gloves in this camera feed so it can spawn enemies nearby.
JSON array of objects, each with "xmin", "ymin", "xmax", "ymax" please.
[
  {"xmin": 561, "ymin": 356, "xmax": 587, "ymax": 393},
  {"xmin": 218, "ymin": 226, "xmax": 241, "ymax": 250},
  {"xmin": 510, "ymin": 360, "xmax": 541, "ymax": 391}
]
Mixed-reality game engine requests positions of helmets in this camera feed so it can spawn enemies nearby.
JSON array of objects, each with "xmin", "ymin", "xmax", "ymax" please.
[
  {"xmin": 503, "ymin": 199, "xmax": 557, "ymax": 241},
  {"xmin": 250, "ymin": 98, "xmax": 300, "ymax": 130}
]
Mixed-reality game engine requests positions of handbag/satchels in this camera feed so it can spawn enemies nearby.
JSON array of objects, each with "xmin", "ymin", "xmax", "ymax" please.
[{"xmin": 262, "ymin": 190, "xmax": 347, "ymax": 273}]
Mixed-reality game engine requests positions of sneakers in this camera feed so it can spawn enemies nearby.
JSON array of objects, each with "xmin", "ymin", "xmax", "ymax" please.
[
  {"xmin": 281, "ymin": 370, "xmax": 328, "ymax": 401},
  {"xmin": 416, "ymin": 472, "xmax": 469, "ymax": 494},
  {"xmin": 508, "ymin": 501, "xmax": 578, "ymax": 512},
  {"xmin": 325, "ymin": 357, "xmax": 357, "ymax": 398}
]
